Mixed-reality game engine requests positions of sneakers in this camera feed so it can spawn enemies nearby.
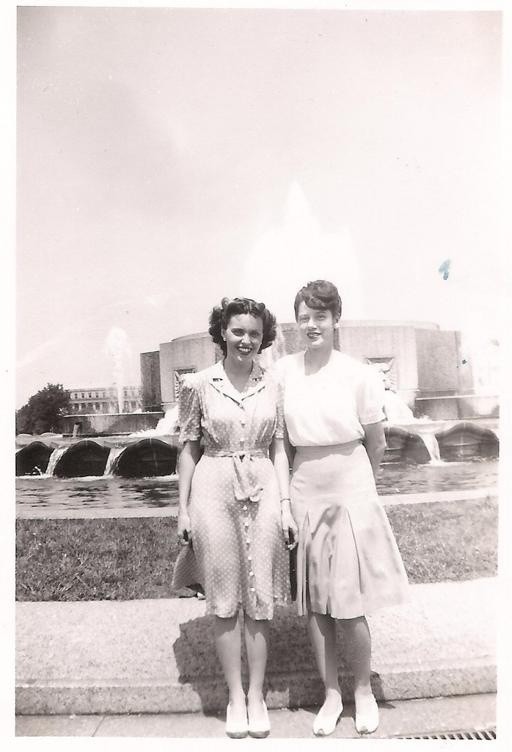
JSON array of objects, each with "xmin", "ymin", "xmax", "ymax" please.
[
  {"xmin": 226, "ymin": 703, "xmax": 248, "ymax": 738},
  {"xmin": 355, "ymin": 694, "xmax": 379, "ymax": 734},
  {"xmin": 312, "ymin": 703, "xmax": 343, "ymax": 736},
  {"xmin": 248, "ymin": 700, "xmax": 270, "ymax": 738}
]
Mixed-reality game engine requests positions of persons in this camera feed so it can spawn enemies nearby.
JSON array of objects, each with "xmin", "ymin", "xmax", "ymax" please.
[
  {"xmin": 275, "ymin": 278, "xmax": 410, "ymax": 735},
  {"xmin": 173, "ymin": 296, "xmax": 299, "ymax": 739}
]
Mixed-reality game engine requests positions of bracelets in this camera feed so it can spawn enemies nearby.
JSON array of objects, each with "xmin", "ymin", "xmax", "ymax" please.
[{"xmin": 280, "ymin": 496, "xmax": 290, "ymax": 503}]
